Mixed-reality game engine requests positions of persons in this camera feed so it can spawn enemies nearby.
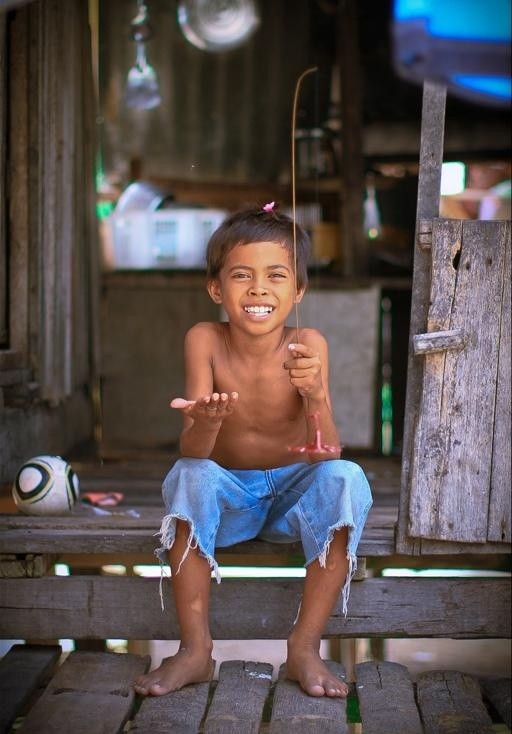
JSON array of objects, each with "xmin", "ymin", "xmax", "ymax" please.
[{"xmin": 130, "ymin": 208, "xmax": 374, "ymax": 699}]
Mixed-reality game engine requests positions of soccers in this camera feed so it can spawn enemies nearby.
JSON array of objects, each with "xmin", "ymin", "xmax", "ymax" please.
[{"xmin": 12, "ymin": 455, "xmax": 80, "ymax": 516}]
